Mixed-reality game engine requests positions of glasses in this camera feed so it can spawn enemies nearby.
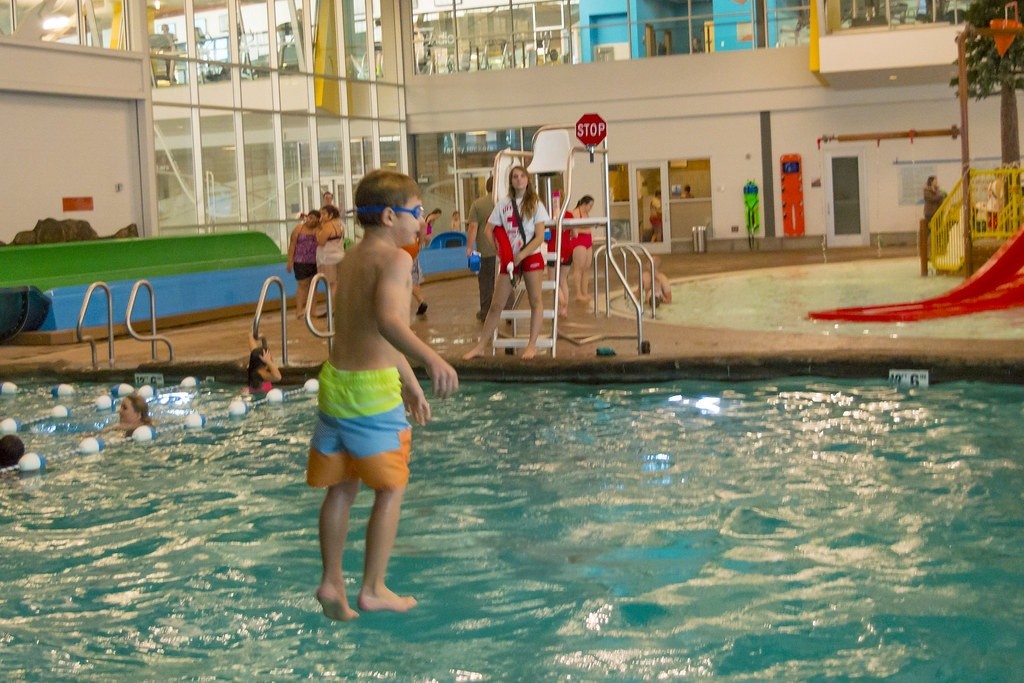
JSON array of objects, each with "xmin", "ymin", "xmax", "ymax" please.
[{"xmin": 391, "ymin": 205, "xmax": 426, "ymax": 220}]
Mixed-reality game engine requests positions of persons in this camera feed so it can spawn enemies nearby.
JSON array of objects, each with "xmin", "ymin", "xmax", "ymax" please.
[
  {"xmin": 547, "ymin": 196, "xmax": 594, "ymax": 317},
  {"xmin": 465, "ymin": 176, "xmax": 515, "ymax": 323},
  {"xmin": 247, "ymin": 331, "xmax": 281, "ymax": 393},
  {"xmin": 684, "ymin": 186, "xmax": 694, "ymax": 198},
  {"xmin": 322, "ymin": 191, "xmax": 333, "ymax": 204},
  {"xmin": 400, "ymin": 217, "xmax": 429, "ymax": 314},
  {"xmin": 649, "ymin": 191, "xmax": 663, "ymax": 242},
  {"xmin": 924, "ymin": 176, "xmax": 947, "ymax": 221},
  {"xmin": 0, "ymin": 435, "xmax": 25, "ymax": 468},
  {"xmin": 452, "ymin": 212, "xmax": 460, "ymax": 231},
  {"xmin": 462, "ymin": 166, "xmax": 551, "ymax": 361},
  {"xmin": 308, "ymin": 168, "xmax": 459, "ymax": 623},
  {"xmin": 424, "ymin": 208, "xmax": 442, "ymax": 241},
  {"xmin": 987, "ymin": 167, "xmax": 1002, "ymax": 232},
  {"xmin": 119, "ymin": 394, "xmax": 150, "ymax": 424},
  {"xmin": 634, "ymin": 255, "xmax": 672, "ymax": 304},
  {"xmin": 285, "ymin": 204, "xmax": 346, "ymax": 317},
  {"xmin": 161, "ymin": 24, "xmax": 178, "ymax": 81}
]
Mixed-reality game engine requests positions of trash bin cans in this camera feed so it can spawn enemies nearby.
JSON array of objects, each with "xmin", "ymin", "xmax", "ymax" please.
[{"xmin": 691, "ymin": 226, "xmax": 707, "ymax": 253}]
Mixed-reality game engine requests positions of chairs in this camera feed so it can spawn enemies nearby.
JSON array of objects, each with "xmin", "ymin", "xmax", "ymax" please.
[{"xmin": 526, "ymin": 129, "xmax": 573, "ymax": 174}]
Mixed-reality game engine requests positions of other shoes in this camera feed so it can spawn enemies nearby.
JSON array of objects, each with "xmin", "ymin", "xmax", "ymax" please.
[{"xmin": 416, "ymin": 301, "xmax": 428, "ymax": 316}]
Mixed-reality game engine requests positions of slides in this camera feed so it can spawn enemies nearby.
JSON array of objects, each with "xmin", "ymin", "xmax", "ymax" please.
[{"xmin": 808, "ymin": 223, "xmax": 1024, "ymax": 322}]
